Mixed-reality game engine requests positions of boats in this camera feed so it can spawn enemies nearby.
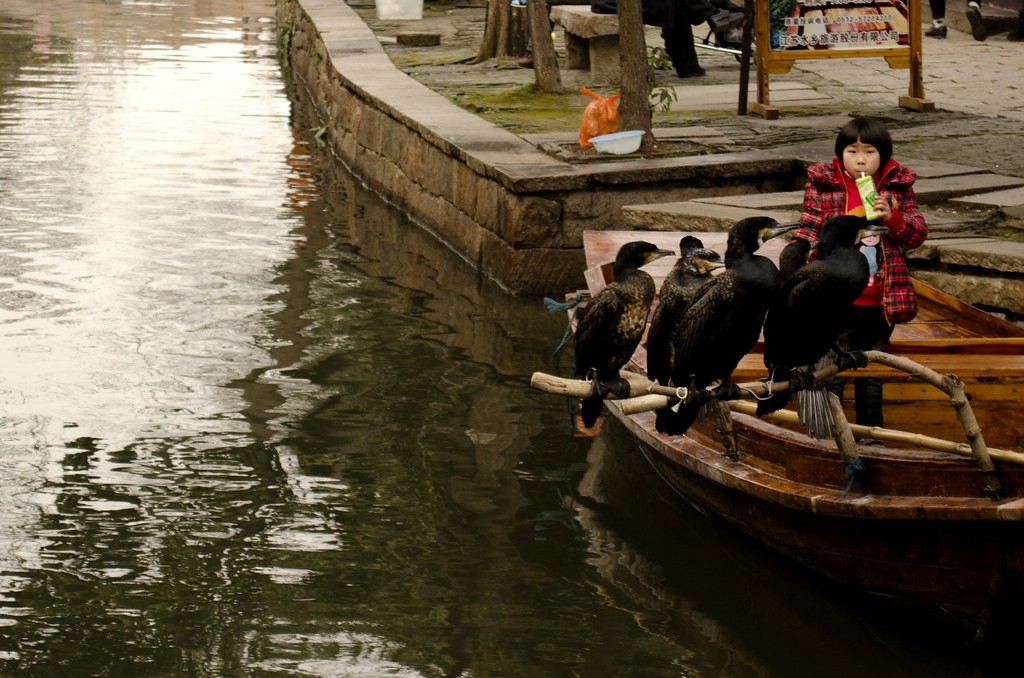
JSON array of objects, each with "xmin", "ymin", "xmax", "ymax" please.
[{"xmin": 529, "ymin": 227, "xmax": 1023, "ymax": 613}]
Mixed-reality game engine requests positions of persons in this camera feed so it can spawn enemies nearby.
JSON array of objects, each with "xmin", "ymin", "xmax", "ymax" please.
[
  {"xmin": 924, "ymin": 0, "xmax": 986, "ymax": 41},
  {"xmin": 510, "ymin": 0, "xmax": 744, "ymax": 77},
  {"xmin": 790, "ymin": 115, "xmax": 928, "ymax": 447}
]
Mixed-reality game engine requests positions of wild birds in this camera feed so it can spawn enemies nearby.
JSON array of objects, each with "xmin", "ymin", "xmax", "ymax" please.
[{"xmin": 566, "ymin": 214, "xmax": 890, "ymax": 437}]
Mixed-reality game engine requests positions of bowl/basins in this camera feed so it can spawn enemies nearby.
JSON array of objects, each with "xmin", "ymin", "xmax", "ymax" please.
[{"xmin": 587, "ymin": 129, "xmax": 645, "ymax": 155}]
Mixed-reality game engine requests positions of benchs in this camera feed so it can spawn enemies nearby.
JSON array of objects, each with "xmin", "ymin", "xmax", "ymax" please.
[
  {"xmin": 729, "ymin": 336, "xmax": 1024, "ymax": 400},
  {"xmin": 550, "ymin": 5, "xmax": 620, "ymax": 86}
]
